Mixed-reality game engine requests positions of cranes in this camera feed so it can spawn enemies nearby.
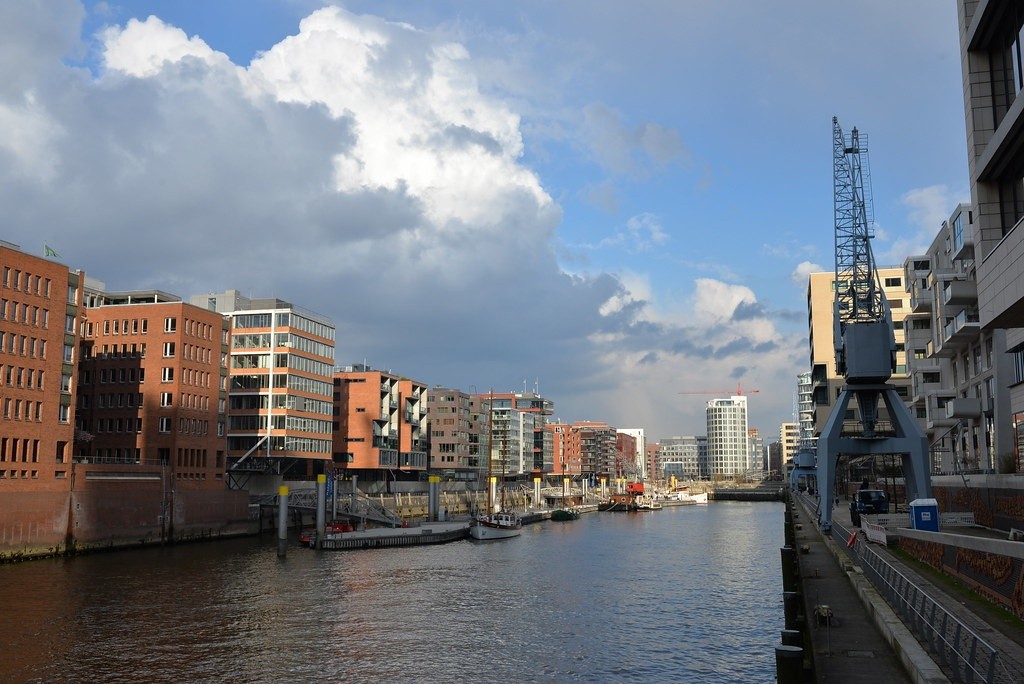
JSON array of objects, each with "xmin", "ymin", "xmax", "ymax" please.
[
  {"xmin": 678, "ymin": 380, "xmax": 761, "ymax": 396},
  {"xmin": 819, "ymin": 113, "xmax": 934, "ymax": 525}
]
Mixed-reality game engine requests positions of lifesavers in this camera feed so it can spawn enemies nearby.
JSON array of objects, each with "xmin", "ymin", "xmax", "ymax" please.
[
  {"xmin": 846, "ymin": 532, "xmax": 857, "ymax": 546},
  {"xmin": 402, "ymin": 520, "xmax": 408, "ymax": 528}
]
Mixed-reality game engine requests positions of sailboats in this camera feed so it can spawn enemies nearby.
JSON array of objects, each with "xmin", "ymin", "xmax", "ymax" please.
[{"xmin": 468, "ymin": 386, "xmax": 523, "ymax": 540}]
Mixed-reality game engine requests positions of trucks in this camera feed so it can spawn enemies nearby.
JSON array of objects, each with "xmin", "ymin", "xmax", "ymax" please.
[{"xmin": 849, "ymin": 489, "xmax": 891, "ymax": 527}]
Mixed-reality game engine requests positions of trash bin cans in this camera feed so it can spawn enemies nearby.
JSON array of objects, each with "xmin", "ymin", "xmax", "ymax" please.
[{"xmin": 912, "ymin": 499, "xmax": 939, "ymax": 531}]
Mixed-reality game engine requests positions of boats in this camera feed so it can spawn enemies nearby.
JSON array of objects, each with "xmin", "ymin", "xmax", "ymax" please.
[
  {"xmin": 299, "ymin": 516, "xmax": 352, "ymax": 548},
  {"xmin": 550, "ymin": 497, "xmax": 579, "ymax": 522},
  {"xmin": 596, "ymin": 473, "xmax": 709, "ymax": 513}
]
